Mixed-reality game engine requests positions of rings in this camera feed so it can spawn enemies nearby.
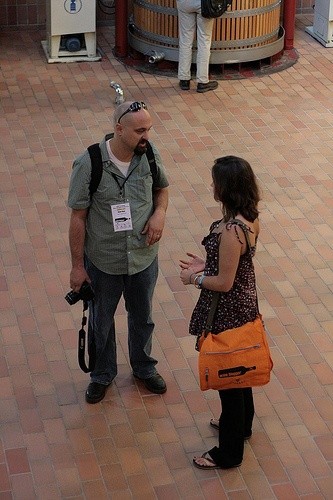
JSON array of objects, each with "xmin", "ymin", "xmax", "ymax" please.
[{"xmin": 157, "ymin": 234, "xmax": 160, "ymax": 237}]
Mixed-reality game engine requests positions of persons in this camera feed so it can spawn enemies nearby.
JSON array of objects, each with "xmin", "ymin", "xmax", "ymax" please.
[
  {"xmin": 176, "ymin": 0, "xmax": 218, "ymax": 92},
  {"xmin": 68, "ymin": 103, "xmax": 169, "ymax": 403},
  {"xmin": 179, "ymin": 156, "xmax": 265, "ymax": 469}
]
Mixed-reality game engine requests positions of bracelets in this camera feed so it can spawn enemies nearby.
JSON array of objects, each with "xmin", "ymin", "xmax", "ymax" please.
[{"xmin": 190, "ymin": 273, "xmax": 205, "ymax": 289}]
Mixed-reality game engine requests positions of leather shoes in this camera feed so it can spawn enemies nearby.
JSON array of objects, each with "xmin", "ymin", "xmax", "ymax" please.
[
  {"xmin": 180, "ymin": 80, "xmax": 190, "ymax": 89},
  {"xmin": 85, "ymin": 381, "xmax": 108, "ymax": 404},
  {"xmin": 196, "ymin": 81, "xmax": 218, "ymax": 92},
  {"xmin": 133, "ymin": 367, "xmax": 167, "ymax": 394}
]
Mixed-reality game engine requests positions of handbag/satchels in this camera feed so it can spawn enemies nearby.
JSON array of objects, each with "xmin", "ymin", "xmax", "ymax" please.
[
  {"xmin": 198, "ymin": 321, "xmax": 274, "ymax": 391},
  {"xmin": 200, "ymin": 0, "xmax": 232, "ymax": 18}
]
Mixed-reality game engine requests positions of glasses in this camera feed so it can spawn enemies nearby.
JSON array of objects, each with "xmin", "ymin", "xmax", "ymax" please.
[{"xmin": 118, "ymin": 102, "xmax": 149, "ymax": 124}]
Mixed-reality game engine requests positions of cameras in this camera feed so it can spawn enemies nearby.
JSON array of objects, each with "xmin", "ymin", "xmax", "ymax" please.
[{"xmin": 64, "ymin": 282, "xmax": 95, "ymax": 306}]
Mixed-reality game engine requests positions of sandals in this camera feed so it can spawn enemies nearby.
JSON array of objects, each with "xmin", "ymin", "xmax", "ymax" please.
[
  {"xmin": 210, "ymin": 419, "xmax": 252, "ymax": 439},
  {"xmin": 193, "ymin": 452, "xmax": 240, "ymax": 469}
]
